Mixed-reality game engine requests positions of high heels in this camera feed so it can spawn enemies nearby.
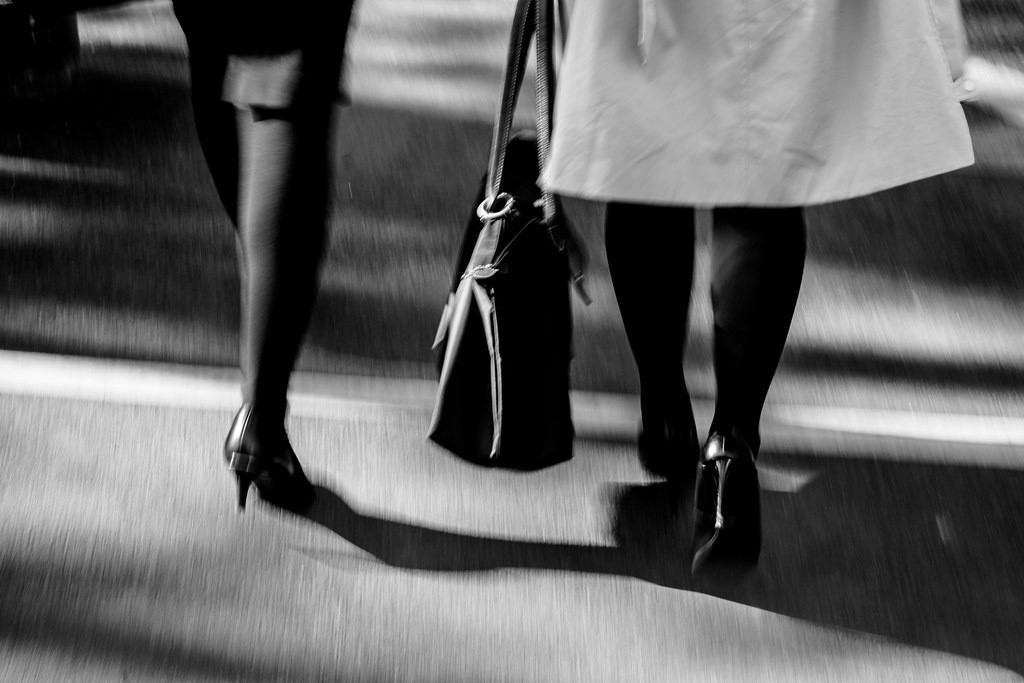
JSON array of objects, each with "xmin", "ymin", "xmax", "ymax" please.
[
  {"xmin": 224, "ymin": 404, "xmax": 316, "ymax": 513},
  {"xmin": 690, "ymin": 428, "xmax": 763, "ymax": 586},
  {"xmin": 635, "ymin": 408, "xmax": 699, "ymax": 483}
]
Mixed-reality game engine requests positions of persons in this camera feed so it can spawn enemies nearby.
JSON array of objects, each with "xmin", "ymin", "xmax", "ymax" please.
[
  {"xmin": 162, "ymin": 1, "xmax": 362, "ymax": 514},
  {"xmin": 533, "ymin": 0, "xmax": 978, "ymax": 594}
]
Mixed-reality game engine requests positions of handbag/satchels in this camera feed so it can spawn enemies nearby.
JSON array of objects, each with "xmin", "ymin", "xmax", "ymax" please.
[{"xmin": 425, "ymin": 0, "xmax": 592, "ymax": 473}]
